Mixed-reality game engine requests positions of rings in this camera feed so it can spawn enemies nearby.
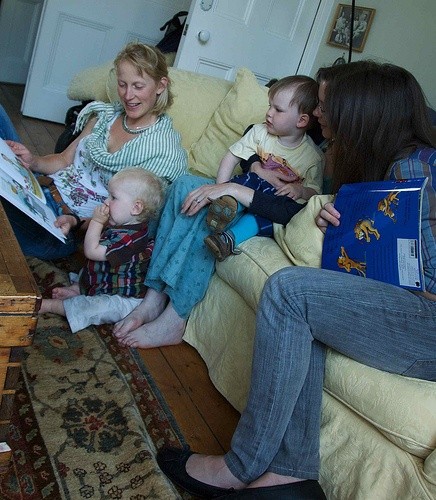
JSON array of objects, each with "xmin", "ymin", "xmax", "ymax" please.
[{"xmin": 194, "ymin": 198, "xmax": 200, "ymax": 203}]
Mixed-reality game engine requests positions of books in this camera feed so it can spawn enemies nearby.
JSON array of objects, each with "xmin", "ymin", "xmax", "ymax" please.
[{"xmin": 322, "ymin": 177, "xmax": 428, "ymax": 293}]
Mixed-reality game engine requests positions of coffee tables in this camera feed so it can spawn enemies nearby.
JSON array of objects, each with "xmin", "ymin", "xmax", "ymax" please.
[{"xmin": 0, "ymin": 199, "xmax": 43, "ymax": 477}]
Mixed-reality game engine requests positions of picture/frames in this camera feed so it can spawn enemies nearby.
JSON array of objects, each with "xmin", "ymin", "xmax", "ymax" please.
[{"xmin": 326, "ymin": 3, "xmax": 377, "ymax": 52}]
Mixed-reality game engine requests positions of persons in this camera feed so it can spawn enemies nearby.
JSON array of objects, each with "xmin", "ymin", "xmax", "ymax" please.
[
  {"xmin": 204, "ymin": 75, "xmax": 325, "ymax": 262},
  {"xmin": 0, "ymin": 41, "xmax": 189, "ymax": 264},
  {"xmin": 155, "ymin": 60, "xmax": 436, "ymax": 500},
  {"xmin": 39, "ymin": 166, "xmax": 168, "ymax": 334},
  {"xmin": 112, "ymin": 66, "xmax": 340, "ymax": 348}
]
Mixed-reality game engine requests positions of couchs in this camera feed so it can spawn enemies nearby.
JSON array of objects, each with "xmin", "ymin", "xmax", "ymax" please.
[{"xmin": 60, "ymin": 64, "xmax": 436, "ymax": 500}]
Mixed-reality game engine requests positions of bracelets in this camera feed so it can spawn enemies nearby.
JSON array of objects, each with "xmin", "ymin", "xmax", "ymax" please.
[{"xmin": 66, "ymin": 213, "xmax": 86, "ymax": 230}]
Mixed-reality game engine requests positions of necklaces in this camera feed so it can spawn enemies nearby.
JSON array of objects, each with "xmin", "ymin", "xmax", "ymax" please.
[{"xmin": 122, "ymin": 112, "xmax": 161, "ymax": 134}]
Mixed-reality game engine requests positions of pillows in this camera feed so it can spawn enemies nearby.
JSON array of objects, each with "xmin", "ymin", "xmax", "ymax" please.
[
  {"xmin": 272, "ymin": 189, "xmax": 339, "ymax": 268},
  {"xmin": 188, "ymin": 66, "xmax": 271, "ymax": 176}
]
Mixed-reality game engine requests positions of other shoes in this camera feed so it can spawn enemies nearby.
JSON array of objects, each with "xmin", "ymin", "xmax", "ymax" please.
[
  {"xmin": 156, "ymin": 445, "xmax": 326, "ymax": 500},
  {"xmin": 206, "ymin": 194, "xmax": 238, "ymax": 234},
  {"xmin": 205, "ymin": 232, "xmax": 242, "ymax": 262}
]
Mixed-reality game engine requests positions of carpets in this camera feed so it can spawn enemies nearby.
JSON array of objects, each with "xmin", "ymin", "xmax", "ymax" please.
[{"xmin": 0, "ymin": 233, "xmax": 197, "ymax": 500}]
